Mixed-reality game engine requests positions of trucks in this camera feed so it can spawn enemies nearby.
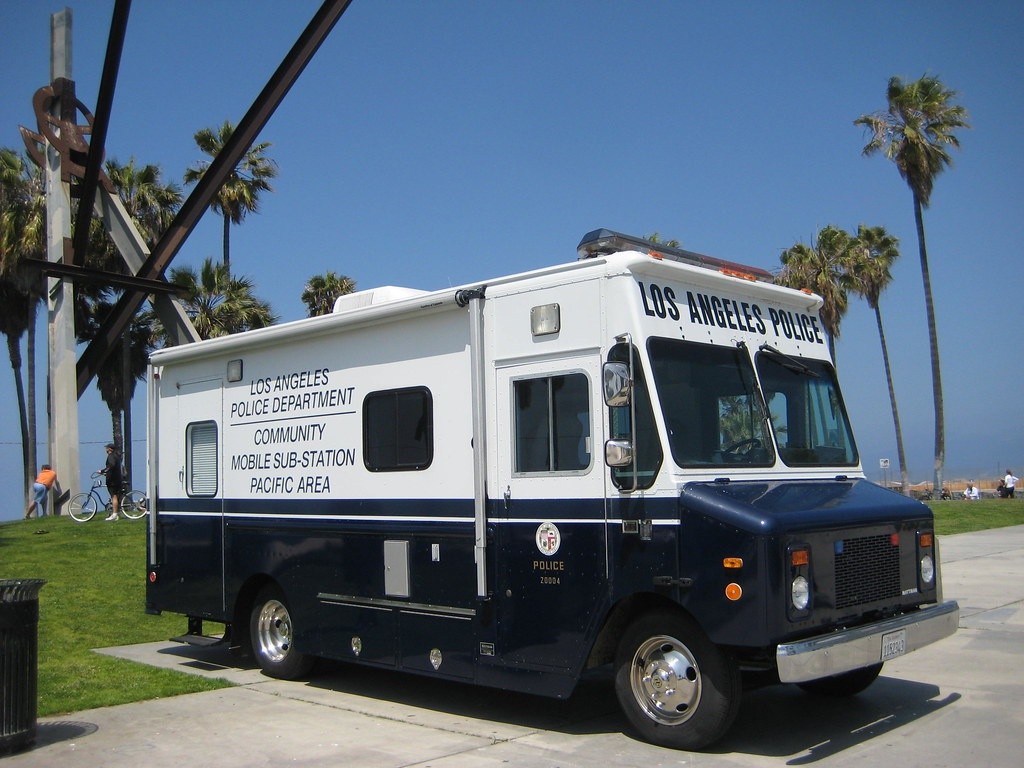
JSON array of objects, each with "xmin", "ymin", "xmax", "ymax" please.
[{"xmin": 142, "ymin": 234, "xmax": 961, "ymax": 751}]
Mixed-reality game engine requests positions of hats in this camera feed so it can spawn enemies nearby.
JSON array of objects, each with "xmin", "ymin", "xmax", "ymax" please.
[{"xmin": 104, "ymin": 443, "xmax": 114, "ymax": 448}]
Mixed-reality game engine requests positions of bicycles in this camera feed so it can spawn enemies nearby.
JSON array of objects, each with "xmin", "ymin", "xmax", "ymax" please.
[{"xmin": 68, "ymin": 469, "xmax": 148, "ymax": 522}]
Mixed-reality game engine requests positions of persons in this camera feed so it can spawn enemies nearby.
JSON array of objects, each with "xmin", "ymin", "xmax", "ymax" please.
[
  {"xmin": 98, "ymin": 443, "xmax": 121, "ymax": 521},
  {"xmin": 25, "ymin": 464, "xmax": 58, "ymax": 519},
  {"xmin": 963, "ymin": 469, "xmax": 1020, "ymax": 500}
]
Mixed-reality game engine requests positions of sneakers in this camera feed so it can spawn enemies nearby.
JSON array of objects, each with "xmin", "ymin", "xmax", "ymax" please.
[{"xmin": 105, "ymin": 515, "xmax": 119, "ymax": 521}]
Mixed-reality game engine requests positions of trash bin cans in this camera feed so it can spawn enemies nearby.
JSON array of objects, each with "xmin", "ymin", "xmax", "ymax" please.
[{"xmin": 0, "ymin": 578, "xmax": 49, "ymax": 757}]
